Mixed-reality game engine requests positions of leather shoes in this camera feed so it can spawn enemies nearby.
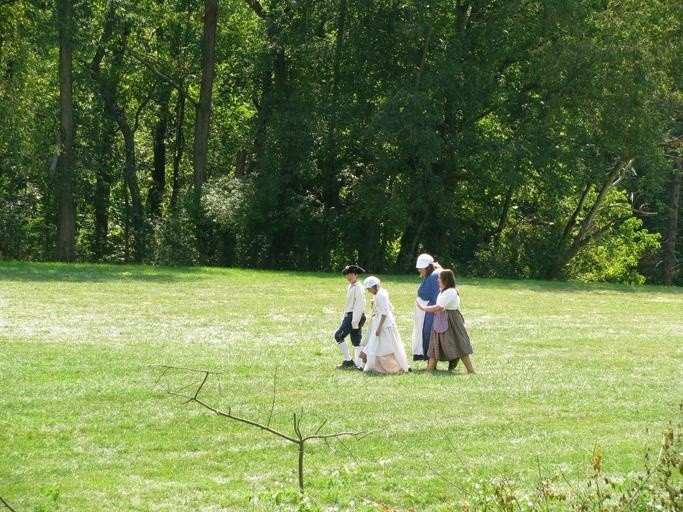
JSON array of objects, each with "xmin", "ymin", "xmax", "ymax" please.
[{"xmin": 336, "ymin": 359, "xmax": 354, "ymax": 369}]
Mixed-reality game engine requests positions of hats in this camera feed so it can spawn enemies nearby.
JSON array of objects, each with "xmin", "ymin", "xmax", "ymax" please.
[
  {"xmin": 415, "ymin": 254, "xmax": 433, "ymax": 269},
  {"xmin": 342, "ymin": 265, "xmax": 365, "ymax": 275},
  {"xmin": 363, "ymin": 276, "xmax": 379, "ymax": 289}
]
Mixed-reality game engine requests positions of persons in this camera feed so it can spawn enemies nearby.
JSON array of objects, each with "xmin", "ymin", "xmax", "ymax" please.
[
  {"xmin": 362, "ymin": 275, "xmax": 409, "ymax": 374},
  {"xmin": 416, "ymin": 269, "xmax": 476, "ymax": 374},
  {"xmin": 412, "ymin": 253, "xmax": 460, "ymax": 371},
  {"xmin": 334, "ymin": 265, "xmax": 367, "ymax": 368}
]
